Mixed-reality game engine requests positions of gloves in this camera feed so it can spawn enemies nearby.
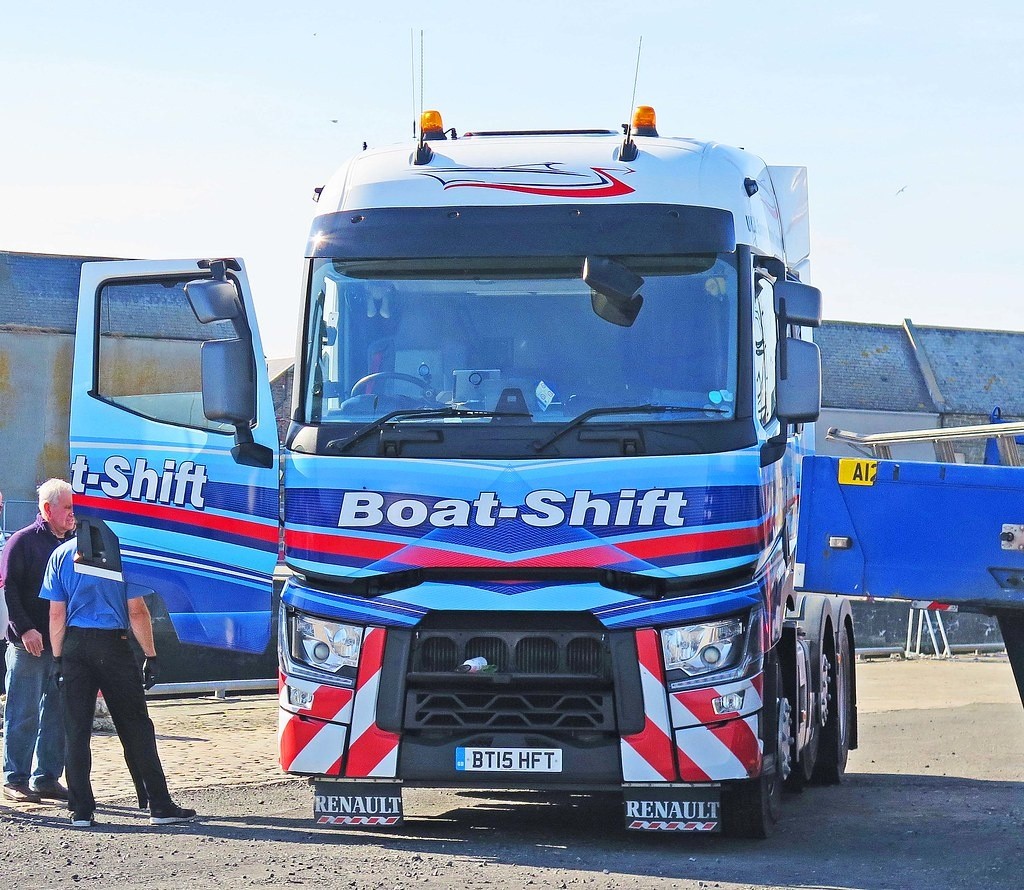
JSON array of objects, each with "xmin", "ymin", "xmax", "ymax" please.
[
  {"xmin": 46, "ymin": 655, "xmax": 63, "ymax": 691},
  {"xmin": 142, "ymin": 654, "xmax": 159, "ymax": 690}
]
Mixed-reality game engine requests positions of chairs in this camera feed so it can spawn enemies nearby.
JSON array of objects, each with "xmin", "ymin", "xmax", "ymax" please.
[{"xmin": 371, "ymin": 295, "xmax": 488, "ymax": 400}]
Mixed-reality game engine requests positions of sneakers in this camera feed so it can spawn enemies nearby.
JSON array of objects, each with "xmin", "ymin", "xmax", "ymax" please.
[
  {"xmin": 3, "ymin": 782, "xmax": 40, "ymax": 803},
  {"xmin": 150, "ymin": 801, "xmax": 196, "ymax": 825},
  {"xmin": 71, "ymin": 811, "xmax": 94, "ymax": 827},
  {"xmin": 33, "ymin": 779, "xmax": 68, "ymax": 799}
]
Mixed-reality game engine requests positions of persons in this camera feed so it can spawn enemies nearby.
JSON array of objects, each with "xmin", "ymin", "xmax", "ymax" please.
[
  {"xmin": 39, "ymin": 537, "xmax": 199, "ymax": 827},
  {"xmin": 0, "ymin": 478, "xmax": 77, "ymax": 804}
]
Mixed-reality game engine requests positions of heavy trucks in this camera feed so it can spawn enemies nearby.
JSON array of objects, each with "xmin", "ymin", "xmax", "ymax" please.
[{"xmin": 63, "ymin": 103, "xmax": 1024, "ymax": 840}]
